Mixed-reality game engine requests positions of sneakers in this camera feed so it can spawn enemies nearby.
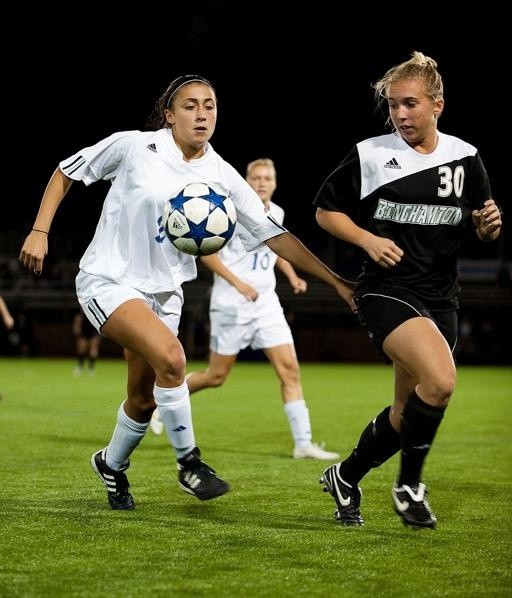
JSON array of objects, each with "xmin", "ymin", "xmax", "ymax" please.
[
  {"xmin": 90, "ymin": 445, "xmax": 137, "ymax": 511},
  {"xmin": 149, "ymin": 406, "xmax": 165, "ymax": 435},
  {"xmin": 390, "ymin": 480, "xmax": 439, "ymax": 531},
  {"xmin": 176, "ymin": 446, "xmax": 231, "ymax": 502},
  {"xmin": 292, "ymin": 441, "xmax": 342, "ymax": 462},
  {"xmin": 318, "ymin": 462, "xmax": 365, "ymax": 527}
]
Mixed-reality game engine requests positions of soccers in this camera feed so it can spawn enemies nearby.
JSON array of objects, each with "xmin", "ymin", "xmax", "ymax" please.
[{"xmin": 164, "ymin": 182, "xmax": 236, "ymax": 255}]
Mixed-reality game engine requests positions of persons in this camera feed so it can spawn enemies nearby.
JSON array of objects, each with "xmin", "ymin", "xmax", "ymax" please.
[
  {"xmin": 17, "ymin": 71, "xmax": 361, "ymax": 513},
  {"xmin": 147, "ymin": 158, "xmax": 341, "ymax": 460},
  {"xmin": 313, "ymin": 50, "xmax": 502, "ymax": 529}
]
